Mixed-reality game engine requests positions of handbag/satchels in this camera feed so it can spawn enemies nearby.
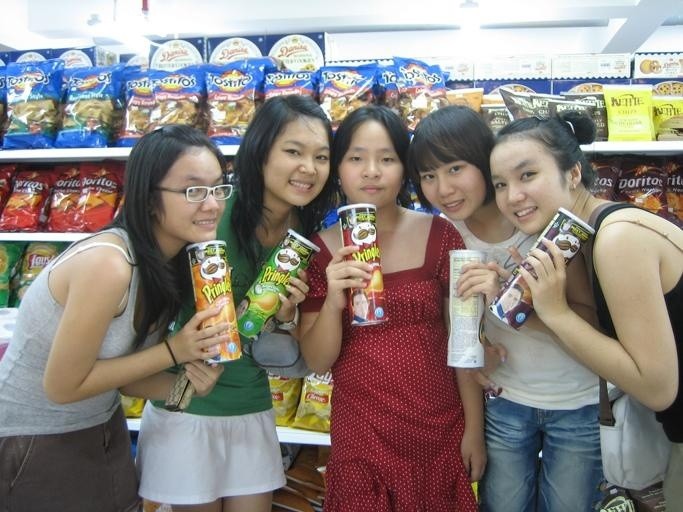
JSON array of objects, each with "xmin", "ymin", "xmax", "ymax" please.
[{"xmin": 599, "ymin": 392, "xmax": 672, "ymax": 492}]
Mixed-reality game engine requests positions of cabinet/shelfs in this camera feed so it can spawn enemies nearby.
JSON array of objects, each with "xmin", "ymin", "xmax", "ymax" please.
[{"xmin": 0, "ymin": 139, "xmax": 682, "ymax": 512}]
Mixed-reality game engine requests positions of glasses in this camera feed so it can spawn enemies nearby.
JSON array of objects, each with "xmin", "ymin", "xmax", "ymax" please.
[{"xmin": 152, "ymin": 184, "xmax": 233, "ymax": 203}]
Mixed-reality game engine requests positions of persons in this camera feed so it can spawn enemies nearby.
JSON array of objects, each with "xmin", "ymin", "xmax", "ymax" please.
[
  {"xmin": 0, "ymin": 120, "xmax": 232, "ymax": 510},
  {"xmin": 496, "ymin": 284, "xmax": 526, "ymax": 325},
  {"xmin": 294, "ymin": 102, "xmax": 488, "ymax": 511},
  {"xmin": 350, "ymin": 290, "xmax": 369, "ymax": 326},
  {"xmin": 490, "ymin": 109, "xmax": 682, "ymax": 511},
  {"xmin": 235, "ymin": 297, "xmax": 249, "ymax": 320},
  {"xmin": 202, "ymin": 325, "xmax": 228, "ymax": 363},
  {"xmin": 130, "ymin": 92, "xmax": 334, "ymax": 511},
  {"xmin": 407, "ymin": 105, "xmax": 627, "ymax": 511}
]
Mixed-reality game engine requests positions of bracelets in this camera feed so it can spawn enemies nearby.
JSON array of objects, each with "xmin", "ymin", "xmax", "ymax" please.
[{"xmin": 162, "ymin": 340, "xmax": 179, "ymax": 368}]
